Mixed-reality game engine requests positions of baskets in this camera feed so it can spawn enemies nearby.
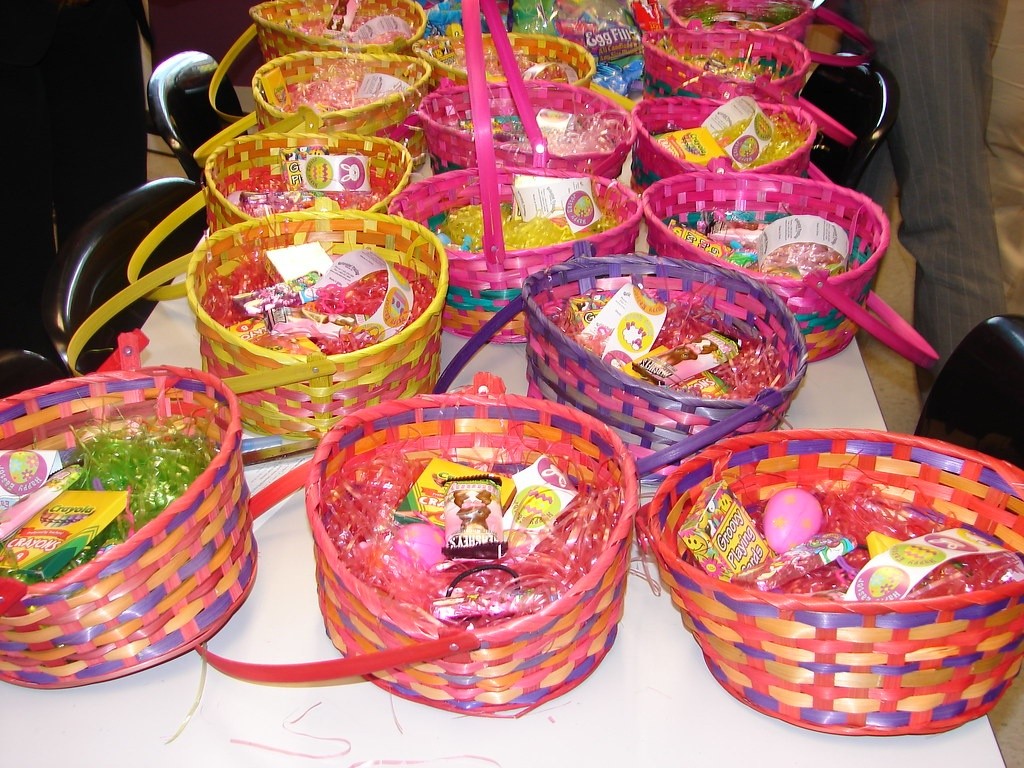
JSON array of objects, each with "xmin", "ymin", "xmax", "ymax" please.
[
  {"xmin": 193, "ymin": 393, "xmax": 632, "ymax": 717},
  {"xmin": 644, "ymin": 426, "xmax": 1023, "ymax": 735},
  {"xmin": 196, "ymin": 50, "xmax": 430, "ymax": 168},
  {"xmin": 130, "ymin": 131, "xmax": 413, "ymax": 301},
  {"xmin": 633, "ymin": 95, "xmax": 836, "ymax": 198},
  {"xmin": 0, "ymin": 368, "xmax": 257, "ymax": 689},
  {"xmin": 420, "ymin": 78, "xmax": 637, "ymax": 182},
  {"xmin": 668, "ymin": 0, "xmax": 876, "ymax": 67},
  {"xmin": 207, "ymin": 2, "xmax": 425, "ymax": 124},
  {"xmin": 67, "ymin": 208, "xmax": 451, "ymax": 442},
  {"xmin": 644, "ymin": 170, "xmax": 940, "ymax": 367},
  {"xmin": 388, "ymin": 166, "xmax": 644, "ymax": 343},
  {"xmin": 437, "ymin": 255, "xmax": 806, "ymax": 479},
  {"xmin": 411, "ymin": 32, "xmax": 596, "ymax": 93},
  {"xmin": 642, "ymin": 27, "xmax": 856, "ymax": 146}
]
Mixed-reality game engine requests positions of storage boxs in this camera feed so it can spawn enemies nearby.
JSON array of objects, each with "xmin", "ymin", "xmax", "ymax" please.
[{"xmin": 0, "ymin": 0, "xmax": 1024, "ymax": 738}]
